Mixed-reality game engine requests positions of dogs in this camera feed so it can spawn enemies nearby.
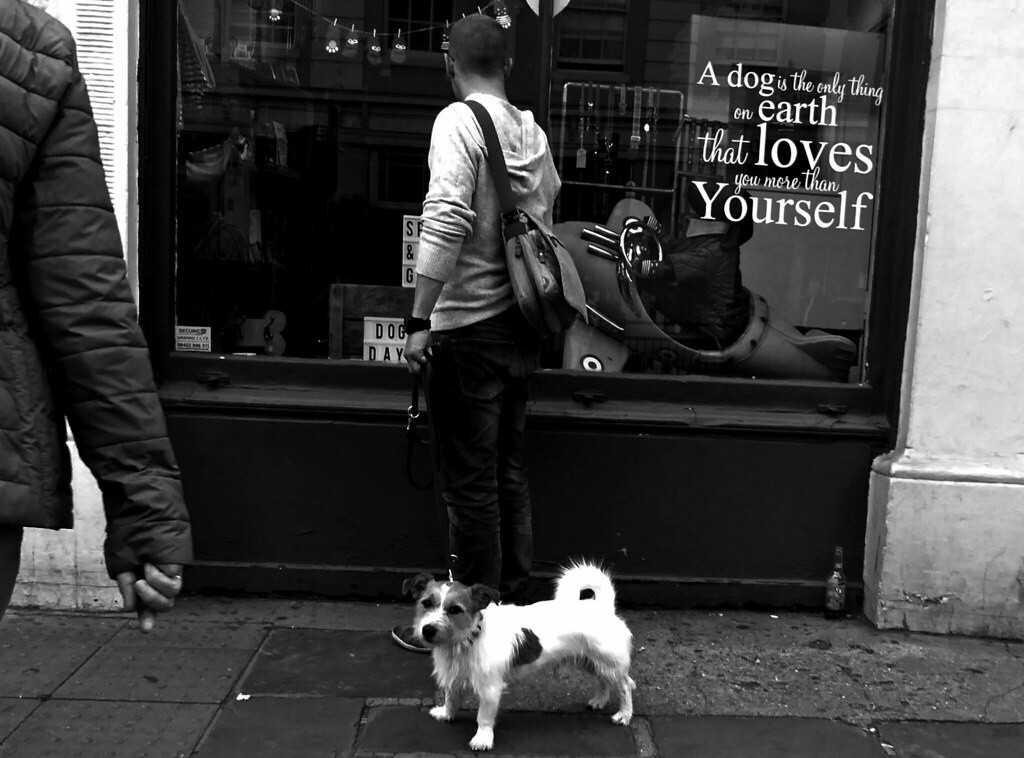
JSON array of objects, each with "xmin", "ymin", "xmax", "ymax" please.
[{"xmin": 400, "ymin": 563, "xmax": 635, "ymax": 751}]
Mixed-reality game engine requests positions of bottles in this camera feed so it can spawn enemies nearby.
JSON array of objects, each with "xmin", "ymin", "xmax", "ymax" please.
[{"xmin": 825, "ymin": 547, "xmax": 846, "ymax": 619}]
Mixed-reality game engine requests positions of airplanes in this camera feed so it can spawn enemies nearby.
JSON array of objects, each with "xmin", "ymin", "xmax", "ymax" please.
[{"xmin": 547, "ymin": 197, "xmax": 857, "ymax": 380}]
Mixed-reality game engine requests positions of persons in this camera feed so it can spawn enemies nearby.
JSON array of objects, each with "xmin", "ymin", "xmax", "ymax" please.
[
  {"xmin": 1, "ymin": 0, "xmax": 195, "ymax": 647},
  {"xmin": 392, "ymin": 12, "xmax": 563, "ymax": 655}
]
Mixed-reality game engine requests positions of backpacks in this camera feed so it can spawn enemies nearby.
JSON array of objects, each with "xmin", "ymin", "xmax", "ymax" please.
[{"xmin": 462, "ymin": 101, "xmax": 588, "ymax": 337}]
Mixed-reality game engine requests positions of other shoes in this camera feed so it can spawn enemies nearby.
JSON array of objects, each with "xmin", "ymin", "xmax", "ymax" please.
[{"xmin": 391, "ymin": 624, "xmax": 434, "ymax": 651}]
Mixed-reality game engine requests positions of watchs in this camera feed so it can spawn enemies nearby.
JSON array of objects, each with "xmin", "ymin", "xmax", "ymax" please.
[{"xmin": 403, "ymin": 315, "xmax": 432, "ymax": 336}]
